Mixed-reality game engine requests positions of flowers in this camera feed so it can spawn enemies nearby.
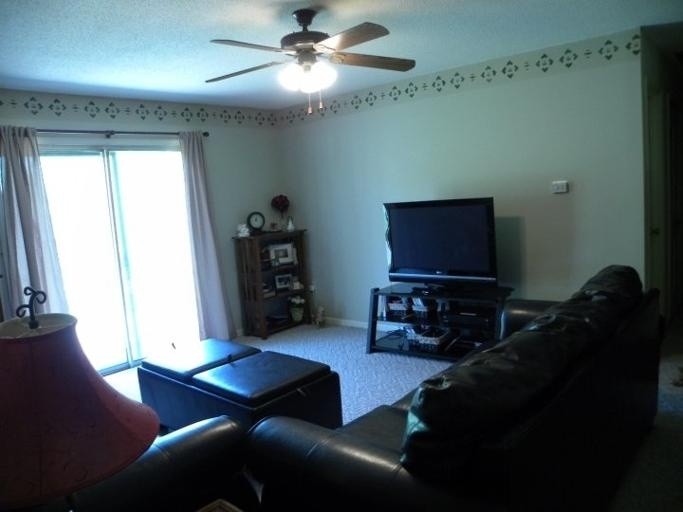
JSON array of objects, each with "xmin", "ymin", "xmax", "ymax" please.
[
  {"xmin": 271, "ymin": 194, "xmax": 289, "ymax": 218},
  {"xmin": 287, "ymin": 294, "xmax": 307, "ymax": 308}
]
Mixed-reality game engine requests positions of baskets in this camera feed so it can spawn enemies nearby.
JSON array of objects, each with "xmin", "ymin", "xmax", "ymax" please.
[{"xmin": 419, "ymin": 329, "xmax": 448, "ymax": 346}]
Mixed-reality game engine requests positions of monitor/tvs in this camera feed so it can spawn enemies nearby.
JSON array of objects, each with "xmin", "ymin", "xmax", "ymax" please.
[{"xmin": 382, "ymin": 197, "xmax": 497, "ymax": 292}]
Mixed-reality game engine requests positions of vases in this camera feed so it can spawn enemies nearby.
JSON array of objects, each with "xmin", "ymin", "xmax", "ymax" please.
[
  {"xmin": 280, "ymin": 217, "xmax": 286, "ymax": 231},
  {"xmin": 286, "ymin": 217, "xmax": 295, "ymax": 232},
  {"xmin": 290, "ymin": 307, "xmax": 304, "ymax": 324}
]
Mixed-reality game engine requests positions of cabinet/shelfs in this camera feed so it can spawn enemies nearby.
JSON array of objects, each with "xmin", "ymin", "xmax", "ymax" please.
[{"xmin": 233, "ymin": 231, "xmax": 313, "ymax": 339}]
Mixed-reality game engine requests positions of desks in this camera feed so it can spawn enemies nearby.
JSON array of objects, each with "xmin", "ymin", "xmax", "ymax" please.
[{"xmin": 365, "ymin": 282, "xmax": 514, "ymax": 362}]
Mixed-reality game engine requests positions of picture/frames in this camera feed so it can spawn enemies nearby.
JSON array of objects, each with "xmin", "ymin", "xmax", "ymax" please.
[
  {"xmin": 274, "ymin": 273, "xmax": 293, "ymax": 291},
  {"xmin": 269, "ymin": 243, "xmax": 293, "ymax": 265}
]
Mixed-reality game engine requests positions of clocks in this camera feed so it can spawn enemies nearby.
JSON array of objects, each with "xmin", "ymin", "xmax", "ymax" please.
[{"xmin": 247, "ymin": 212, "xmax": 266, "ymax": 236}]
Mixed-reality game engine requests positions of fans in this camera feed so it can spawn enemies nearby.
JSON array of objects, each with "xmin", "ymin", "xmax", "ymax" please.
[{"xmin": 205, "ymin": 10, "xmax": 416, "ymax": 115}]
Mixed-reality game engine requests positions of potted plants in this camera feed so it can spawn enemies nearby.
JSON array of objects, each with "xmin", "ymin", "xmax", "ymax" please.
[{"xmin": 314, "ymin": 305, "xmax": 327, "ymax": 329}]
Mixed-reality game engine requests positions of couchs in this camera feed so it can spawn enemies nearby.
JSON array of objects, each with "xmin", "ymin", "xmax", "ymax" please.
[
  {"xmin": 70, "ymin": 413, "xmax": 259, "ymax": 511},
  {"xmin": 244, "ymin": 264, "xmax": 666, "ymax": 512}
]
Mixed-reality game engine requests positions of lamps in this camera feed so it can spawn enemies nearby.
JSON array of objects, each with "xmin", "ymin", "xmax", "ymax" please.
[
  {"xmin": 0, "ymin": 285, "xmax": 160, "ymax": 512},
  {"xmin": 276, "ymin": 49, "xmax": 337, "ymax": 114}
]
